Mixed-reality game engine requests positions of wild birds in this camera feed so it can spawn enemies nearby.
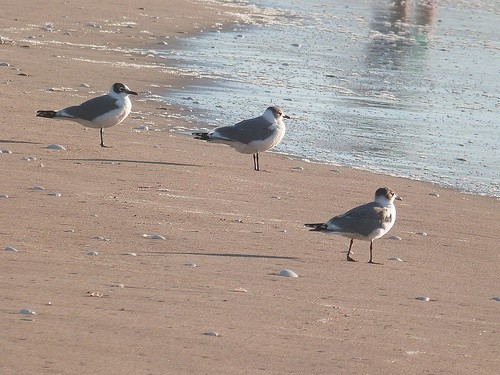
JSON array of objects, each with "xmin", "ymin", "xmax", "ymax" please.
[
  {"xmin": 304, "ymin": 187, "xmax": 403, "ymax": 266},
  {"xmin": 191, "ymin": 106, "xmax": 291, "ymax": 172},
  {"xmin": 36, "ymin": 83, "xmax": 138, "ymax": 148}
]
